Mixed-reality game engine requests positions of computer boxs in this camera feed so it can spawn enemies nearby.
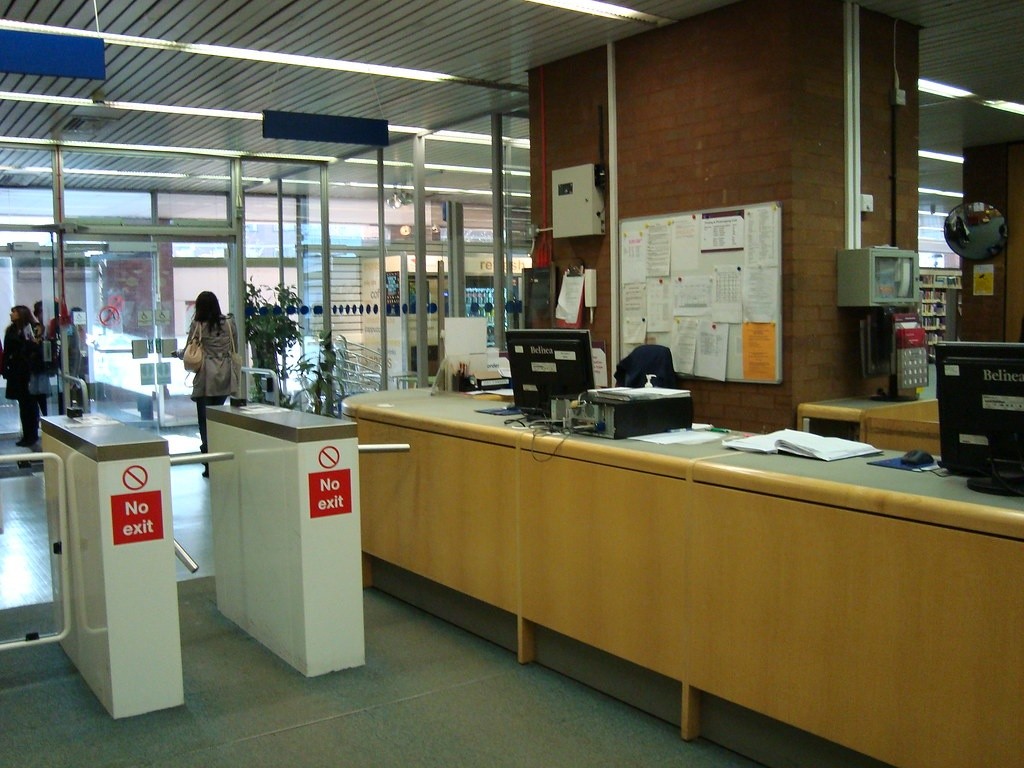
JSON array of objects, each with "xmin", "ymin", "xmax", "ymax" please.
[{"xmin": 568, "ymin": 396, "xmax": 694, "ymax": 440}]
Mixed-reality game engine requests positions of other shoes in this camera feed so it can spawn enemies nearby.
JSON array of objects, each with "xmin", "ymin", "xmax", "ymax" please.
[
  {"xmin": 16, "ymin": 435, "xmax": 39, "ymax": 447},
  {"xmin": 202, "ymin": 470, "xmax": 209, "ymax": 477}
]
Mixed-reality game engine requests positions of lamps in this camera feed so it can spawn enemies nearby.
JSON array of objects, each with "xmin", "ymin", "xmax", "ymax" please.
[{"xmin": 387, "ymin": 182, "xmax": 413, "ymax": 210}]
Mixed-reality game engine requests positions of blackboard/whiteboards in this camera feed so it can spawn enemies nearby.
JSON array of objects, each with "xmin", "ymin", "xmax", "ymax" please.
[{"xmin": 617, "ymin": 204, "xmax": 783, "ymax": 384}]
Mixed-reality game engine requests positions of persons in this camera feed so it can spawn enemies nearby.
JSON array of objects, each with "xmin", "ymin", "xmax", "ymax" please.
[
  {"xmin": 0, "ymin": 301, "xmax": 91, "ymax": 446},
  {"xmin": 171, "ymin": 291, "xmax": 237, "ymax": 479}
]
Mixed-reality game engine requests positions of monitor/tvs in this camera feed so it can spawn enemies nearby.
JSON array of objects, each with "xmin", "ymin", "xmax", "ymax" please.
[
  {"xmin": 505, "ymin": 329, "xmax": 595, "ymax": 420},
  {"xmin": 935, "ymin": 340, "xmax": 1024, "ymax": 498}
]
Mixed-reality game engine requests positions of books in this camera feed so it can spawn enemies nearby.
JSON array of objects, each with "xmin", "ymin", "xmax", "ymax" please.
[
  {"xmin": 919, "ymin": 274, "xmax": 961, "ymax": 287},
  {"xmin": 722, "ymin": 427, "xmax": 883, "ymax": 463},
  {"xmin": 922, "ymin": 304, "xmax": 947, "ymax": 314},
  {"xmin": 920, "ymin": 290, "xmax": 947, "ymax": 301},
  {"xmin": 921, "ymin": 316, "xmax": 940, "ymax": 327},
  {"xmin": 927, "ymin": 345, "xmax": 936, "ymax": 355},
  {"xmin": 926, "ymin": 332, "xmax": 943, "ymax": 342}
]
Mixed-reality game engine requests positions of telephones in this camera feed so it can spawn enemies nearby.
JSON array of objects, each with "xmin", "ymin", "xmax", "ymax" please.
[{"xmin": 894, "ymin": 258, "xmax": 911, "ymax": 298}]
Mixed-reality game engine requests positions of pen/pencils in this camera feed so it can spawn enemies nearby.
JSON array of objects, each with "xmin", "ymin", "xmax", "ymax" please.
[
  {"xmin": 450, "ymin": 360, "xmax": 471, "ymax": 376},
  {"xmin": 666, "ymin": 428, "xmax": 692, "ymax": 433},
  {"xmin": 704, "ymin": 428, "xmax": 732, "ymax": 434}
]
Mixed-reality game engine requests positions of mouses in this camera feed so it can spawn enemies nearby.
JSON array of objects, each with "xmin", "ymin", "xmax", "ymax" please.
[{"xmin": 901, "ymin": 449, "xmax": 934, "ymax": 468}]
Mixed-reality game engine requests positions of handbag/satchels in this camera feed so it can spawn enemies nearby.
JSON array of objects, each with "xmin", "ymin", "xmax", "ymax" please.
[
  {"xmin": 183, "ymin": 321, "xmax": 202, "ymax": 373},
  {"xmin": 231, "ymin": 352, "xmax": 242, "ymax": 399}
]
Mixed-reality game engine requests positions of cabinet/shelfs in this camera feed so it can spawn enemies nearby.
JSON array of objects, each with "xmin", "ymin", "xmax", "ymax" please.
[
  {"xmin": 919, "ymin": 267, "xmax": 963, "ymax": 346},
  {"xmin": 795, "ymin": 397, "xmax": 901, "ymax": 443}
]
